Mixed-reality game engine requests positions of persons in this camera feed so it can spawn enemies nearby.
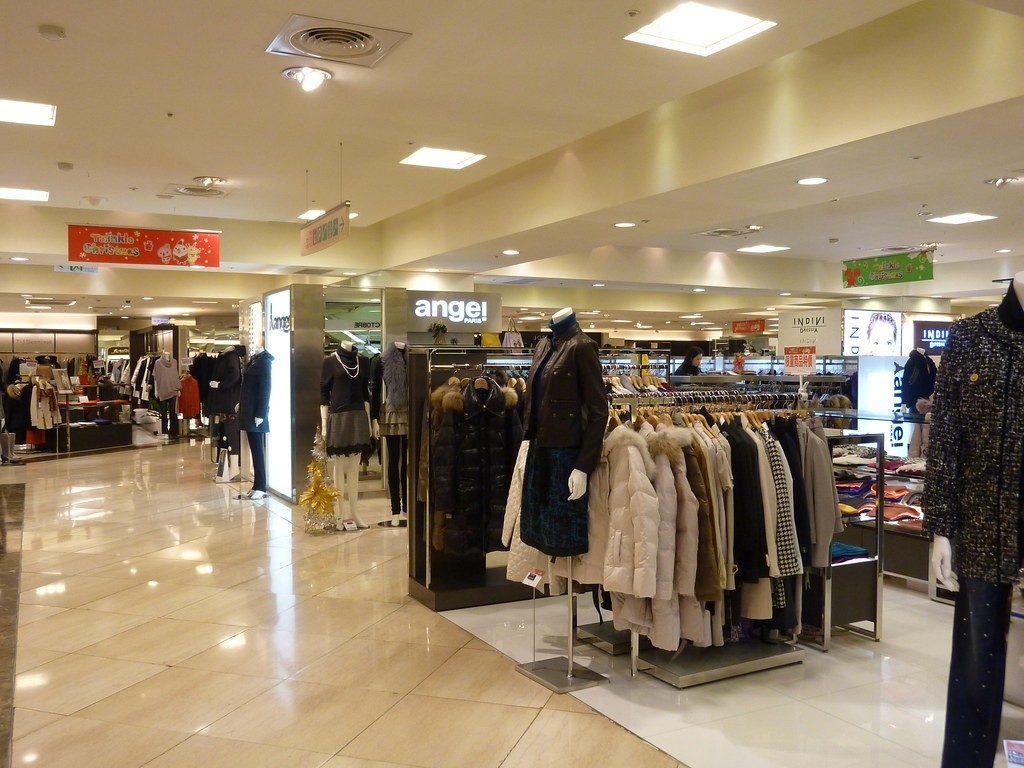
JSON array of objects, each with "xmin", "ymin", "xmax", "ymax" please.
[
  {"xmin": 516, "ymin": 308, "xmax": 608, "ymax": 557},
  {"xmin": 319, "ymin": 341, "xmax": 372, "ymax": 530},
  {"xmin": 152, "ymin": 352, "xmax": 182, "ymax": 435},
  {"xmin": 922, "ymin": 270, "xmax": 1024, "ymax": 768},
  {"xmin": 209, "ymin": 346, "xmax": 246, "ymax": 480},
  {"xmin": 674, "ymin": 346, "xmax": 703, "ymax": 376},
  {"xmin": 240, "ymin": 346, "xmax": 274, "ymax": 498},
  {"xmin": 902, "ymin": 348, "xmax": 938, "ymax": 458},
  {"xmin": 370, "ymin": 341, "xmax": 408, "ymax": 526},
  {"xmin": 868, "ymin": 313, "xmax": 897, "ymax": 355}
]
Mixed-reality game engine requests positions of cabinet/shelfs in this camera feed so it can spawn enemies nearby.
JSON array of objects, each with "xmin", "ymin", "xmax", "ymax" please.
[
  {"xmin": 816, "ymin": 408, "xmax": 930, "ymax": 587},
  {"xmin": 800, "ymin": 432, "xmax": 885, "ymax": 652},
  {"xmin": 56, "ymin": 382, "xmax": 132, "ymax": 452}
]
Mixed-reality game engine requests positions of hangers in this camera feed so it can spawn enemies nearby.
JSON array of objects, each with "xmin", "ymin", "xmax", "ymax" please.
[
  {"xmin": 62, "ymin": 351, "xmax": 94, "ymax": 362},
  {"xmin": 441, "ymin": 364, "xmax": 534, "ymax": 390},
  {"xmin": 600, "ymin": 362, "xmax": 847, "ymax": 432},
  {"xmin": 142, "ymin": 350, "xmax": 164, "ymax": 359}
]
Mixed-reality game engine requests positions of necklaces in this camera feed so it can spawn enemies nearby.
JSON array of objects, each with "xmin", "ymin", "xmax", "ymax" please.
[{"xmin": 335, "ymin": 352, "xmax": 359, "ymax": 378}]
